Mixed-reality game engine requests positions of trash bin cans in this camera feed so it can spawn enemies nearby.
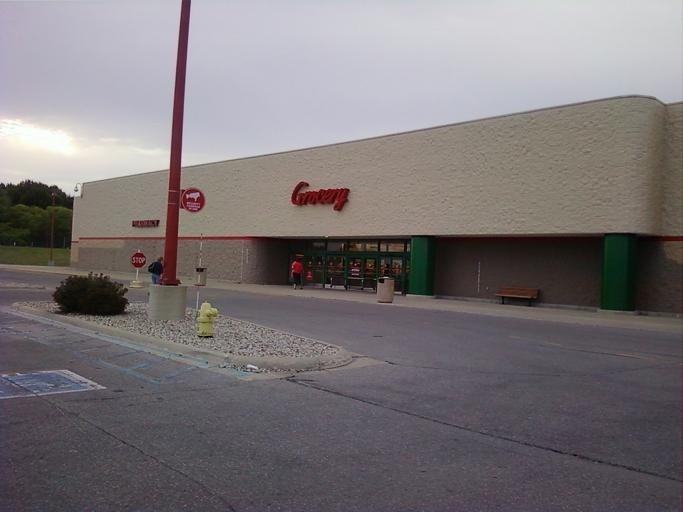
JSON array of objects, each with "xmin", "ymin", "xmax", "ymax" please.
[
  {"xmin": 376, "ymin": 277, "xmax": 394, "ymax": 303},
  {"xmin": 194, "ymin": 267, "xmax": 206, "ymax": 286}
]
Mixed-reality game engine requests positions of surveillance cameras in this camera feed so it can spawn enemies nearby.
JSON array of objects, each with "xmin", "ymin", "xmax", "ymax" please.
[{"xmin": 73, "ymin": 187, "xmax": 78, "ymax": 192}]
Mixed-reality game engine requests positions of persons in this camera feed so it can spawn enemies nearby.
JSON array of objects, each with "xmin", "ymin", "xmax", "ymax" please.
[
  {"xmin": 148, "ymin": 257, "xmax": 163, "ymax": 284},
  {"xmin": 291, "ymin": 258, "xmax": 304, "ymax": 289}
]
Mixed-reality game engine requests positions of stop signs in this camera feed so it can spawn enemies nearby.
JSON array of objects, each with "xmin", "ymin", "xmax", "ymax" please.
[{"xmin": 131, "ymin": 251, "xmax": 146, "ymax": 269}]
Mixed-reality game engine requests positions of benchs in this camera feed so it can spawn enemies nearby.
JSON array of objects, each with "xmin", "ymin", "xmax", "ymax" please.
[{"xmin": 494, "ymin": 286, "xmax": 539, "ymax": 306}]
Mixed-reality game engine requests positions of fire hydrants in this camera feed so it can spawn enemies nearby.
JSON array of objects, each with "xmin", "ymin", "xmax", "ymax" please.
[{"xmin": 195, "ymin": 302, "xmax": 218, "ymax": 339}]
